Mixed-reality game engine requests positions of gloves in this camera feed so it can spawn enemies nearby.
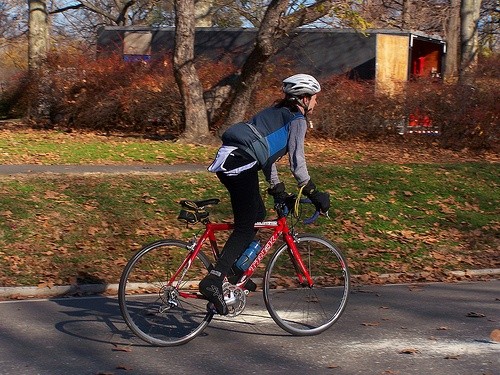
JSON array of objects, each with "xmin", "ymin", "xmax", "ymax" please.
[
  {"xmin": 301, "ymin": 180, "xmax": 330, "ymax": 212},
  {"xmin": 268, "ymin": 182, "xmax": 295, "ymax": 211}
]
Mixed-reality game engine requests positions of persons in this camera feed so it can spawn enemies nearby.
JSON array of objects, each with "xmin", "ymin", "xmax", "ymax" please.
[{"xmin": 198, "ymin": 72, "xmax": 331, "ymax": 316}]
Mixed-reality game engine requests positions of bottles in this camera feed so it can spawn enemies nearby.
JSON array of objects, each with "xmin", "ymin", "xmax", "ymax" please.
[{"xmin": 235, "ymin": 237, "xmax": 263, "ymax": 272}]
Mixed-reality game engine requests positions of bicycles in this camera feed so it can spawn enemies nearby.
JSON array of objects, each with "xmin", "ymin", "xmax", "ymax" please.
[{"xmin": 115, "ymin": 197, "xmax": 350, "ymax": 347}]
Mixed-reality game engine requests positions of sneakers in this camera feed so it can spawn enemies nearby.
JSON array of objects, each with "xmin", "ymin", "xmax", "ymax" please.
[
  {"xmin": 229, "ymin": 270, "xmax": 257, "ymax": 292},
  {"xmin": 199, "ymin": 270, "xmax": 229, "ymax": 315}
]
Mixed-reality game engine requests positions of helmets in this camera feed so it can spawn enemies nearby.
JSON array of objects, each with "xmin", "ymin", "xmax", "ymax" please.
[{"xmin": 281, "ymin": 73, "xmax": 321, "ymax": 96}]
